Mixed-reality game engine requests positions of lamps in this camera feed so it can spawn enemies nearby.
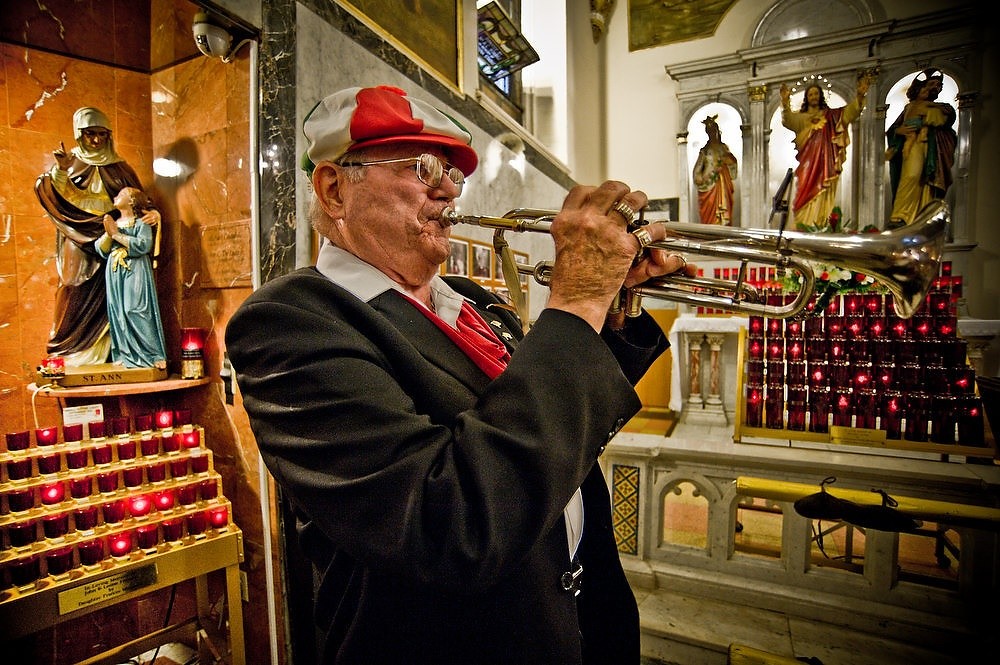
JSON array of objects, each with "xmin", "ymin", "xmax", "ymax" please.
[
  {"xmin": 37, "ymin": 360, "xmax": 65, "ymax": 390},
  {"xmin": 180, "ymin": 327, "xmax": 203, "ymax": 380}
]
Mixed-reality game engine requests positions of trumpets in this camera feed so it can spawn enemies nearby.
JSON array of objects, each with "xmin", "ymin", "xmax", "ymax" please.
[{"xmin": 439, "ymin": 196, "xmax": 955, "ymax": 320}]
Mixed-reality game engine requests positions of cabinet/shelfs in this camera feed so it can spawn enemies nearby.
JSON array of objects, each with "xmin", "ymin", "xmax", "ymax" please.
[{"xmin": 0, "ymin": 423, "xmax": 247, "ymax": 665}]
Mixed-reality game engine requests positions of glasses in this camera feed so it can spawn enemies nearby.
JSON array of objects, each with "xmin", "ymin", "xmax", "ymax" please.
[{"xmin": 338, "ymin": 154, "xmax": 466, "ymax": 198}]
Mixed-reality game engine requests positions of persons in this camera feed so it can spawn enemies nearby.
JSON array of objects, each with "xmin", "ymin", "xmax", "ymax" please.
[
  {"xmin": 223, "ymin": 86, "xmax": 687, "ymax": 664},
  {"xmin": 692, "ymin": 112, "xmax": 743, "ymax": 227},
  {"xmin": 777, "ymin": 72, "xmax": 874, "ymax": 233},
  {"xmin": 93, "ymin": 187, "xmax": 168, "ymax": 370},
  {"xmin": 884, "ymin": 67, "xmax": 959, "ymax": 226},
  {"xmin": 889, "ymin": 82, "xmax": 948, "ymax": 228},
  {"xmin": 32, "ymin": 105, "xmax": 164, "ymax": 367}
]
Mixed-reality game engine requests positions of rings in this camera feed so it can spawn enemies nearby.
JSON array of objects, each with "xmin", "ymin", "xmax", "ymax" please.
[
  {"xmin": 631, "ymin": 228, "xmax": 653, "ymax": 250},
  {"xmin": 613, "ymin": 203, "xmax": 636, "ymax": 226}
]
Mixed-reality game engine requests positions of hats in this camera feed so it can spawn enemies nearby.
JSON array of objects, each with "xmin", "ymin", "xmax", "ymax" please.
[{"xmin": 298, "ymin": 84, "xmax": 478, "ymax": 179}]
